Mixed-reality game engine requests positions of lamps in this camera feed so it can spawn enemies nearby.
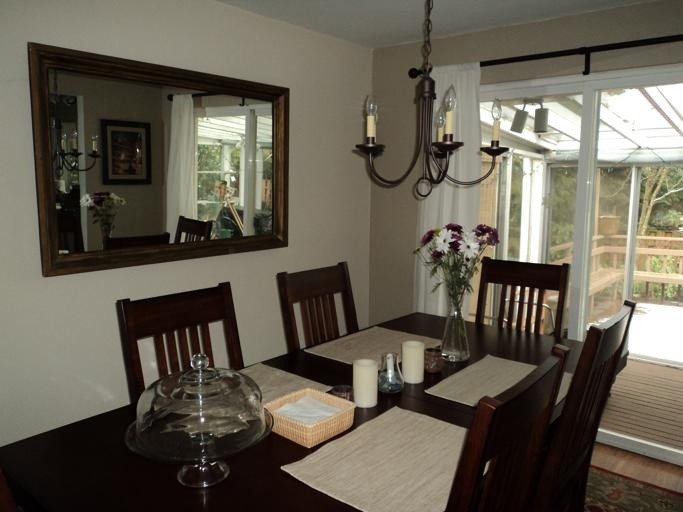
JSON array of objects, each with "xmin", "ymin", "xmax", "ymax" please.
[
  {"xmin": 354, "ymin": 0, "xmax": 509, "ymax": 199},
  {"xmin": 510, "ymin": 98, "xmax": 549, "ymax": 133},
  {"xmin": 50, "ymin": 67, "xmax": 101, "ymax": 177}
]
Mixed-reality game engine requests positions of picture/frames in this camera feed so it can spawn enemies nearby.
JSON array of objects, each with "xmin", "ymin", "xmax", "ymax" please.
[{"xmin": 100, "ymin": 120, "xmax": 152, "ymax": 185}]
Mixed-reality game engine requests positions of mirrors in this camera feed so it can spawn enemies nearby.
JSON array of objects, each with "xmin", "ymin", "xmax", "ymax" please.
[{"xmin": 27, "ymin": 41, "xmax": 288, "ymax": 277}]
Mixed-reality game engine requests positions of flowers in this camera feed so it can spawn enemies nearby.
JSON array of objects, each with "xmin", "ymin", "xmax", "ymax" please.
[
  {"xmin": 80, "ymin": 191, "xmax": 127, "ymax": 234},
  {"xmin": 413, "ymin": 222, "xmax": 499, "ymax": 356}
]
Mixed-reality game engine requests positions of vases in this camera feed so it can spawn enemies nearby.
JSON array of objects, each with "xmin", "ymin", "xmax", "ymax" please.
[
  {"xmin": 101, "ymin": 223, "xmax": 113, "ymax": 251},
  {"xmin": 440, "ymin": 292, "xmax": 471, "ymax": 362}
]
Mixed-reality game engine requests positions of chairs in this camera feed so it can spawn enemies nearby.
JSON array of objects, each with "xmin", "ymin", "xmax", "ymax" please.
[
  {"xmin": 537, "ymin": 297, "xmax": 635, "ymax": 512},
  {"xmin": 276, "ymin": 261, "xmax": 359, "ymax": 354},
  {"xmin": 115, "ymin": 281, "xmax": 244, "ymax": 402},
  {"xmin": 446, "ymin": 343, "xmax": 571, "ymax": 512},
  {"xmin": 175, "ymin": 215, "xmax": 212, "ymax": 244},
  {"xmin": 108, "ymin": 231, "xmax": 170, "ymax": 250},
  {"xmin": 474, "ymin": 256, "xmax": 570, "ymax": 333}
]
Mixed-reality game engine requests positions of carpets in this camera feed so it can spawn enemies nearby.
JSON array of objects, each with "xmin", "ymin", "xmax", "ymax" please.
[{"xmin": 583, "ymin": 464, "xmax": 683, "ymax": 512}]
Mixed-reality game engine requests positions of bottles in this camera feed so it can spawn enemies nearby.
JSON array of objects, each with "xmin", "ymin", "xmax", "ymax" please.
[
  {"xmin": 377, "ymin": 352, "xmax": 405, "ymax": 394},
  {"xmin": 424, "ymin": 348, "xmax": 443, "ymax": 373}
]
